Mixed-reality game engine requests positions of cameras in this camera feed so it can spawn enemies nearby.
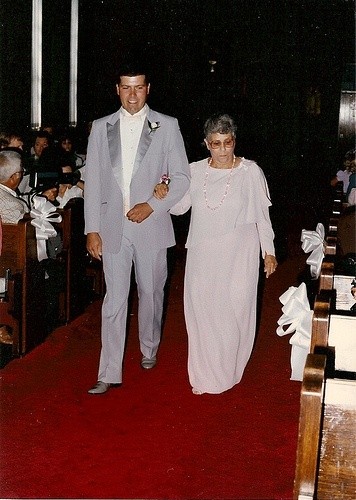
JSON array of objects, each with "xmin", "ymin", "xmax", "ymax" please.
[{"xmin": 29, "ymin": 153, "xmax": 80, "ymax": 190}]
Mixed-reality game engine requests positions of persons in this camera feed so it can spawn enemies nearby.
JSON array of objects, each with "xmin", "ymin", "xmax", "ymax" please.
[
  {"xmin": 330, "ymin": 149, "xmax": 356, "ymax": 206},
  {"xmin": 153, "ymin": 112, "xmax": 278, "ymax": 394},
  {"xmin": 0, "ymin": 126, "xmax": 85, "ymax": 281},
  {"xmin": 84, "ymin": 68, "xmax": 190, "ymax": 394}
]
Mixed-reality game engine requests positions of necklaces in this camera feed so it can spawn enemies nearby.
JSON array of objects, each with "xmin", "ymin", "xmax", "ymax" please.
[{"xmin": 203, "ymin": 153, "xmax": 235, "ymax": 211}]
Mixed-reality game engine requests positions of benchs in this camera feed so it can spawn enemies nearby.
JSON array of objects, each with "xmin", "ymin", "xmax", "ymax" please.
[
  {"xmin": 293, "ymin": 181, "xmax": 356, "ymax": 500},
  {"xmin": 1, "ymin": 203, "xmax": 106, "ymax": 357}
]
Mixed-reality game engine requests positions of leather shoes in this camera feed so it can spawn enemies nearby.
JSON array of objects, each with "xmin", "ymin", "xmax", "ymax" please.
[
  {"xmin": 143, "ymin": 357, "xmax": 158, "ymax": 370},
  {"xmin": 89, "ymin": 381, "xmax": 120, "ymax": 394}
]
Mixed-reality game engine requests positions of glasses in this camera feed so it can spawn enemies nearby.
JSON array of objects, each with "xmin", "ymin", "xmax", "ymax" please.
[
  {"xmin": 10, "ymin": 170, "xmax": 26, "ymax": 178},
  {"xmin": 209, "ymin": 138, "xmax": 234, "ymax": 149}
]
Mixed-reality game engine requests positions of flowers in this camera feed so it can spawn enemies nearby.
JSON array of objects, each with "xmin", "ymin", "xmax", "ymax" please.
[{"xmin": 159, "ymin": 174, "xmax": 171, "ymax": 184}]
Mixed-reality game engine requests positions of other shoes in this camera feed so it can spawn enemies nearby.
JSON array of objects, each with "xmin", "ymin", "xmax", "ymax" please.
[{"xmin": 0, "ymin": 326, "xmax": 14, "ymax": 346}]
